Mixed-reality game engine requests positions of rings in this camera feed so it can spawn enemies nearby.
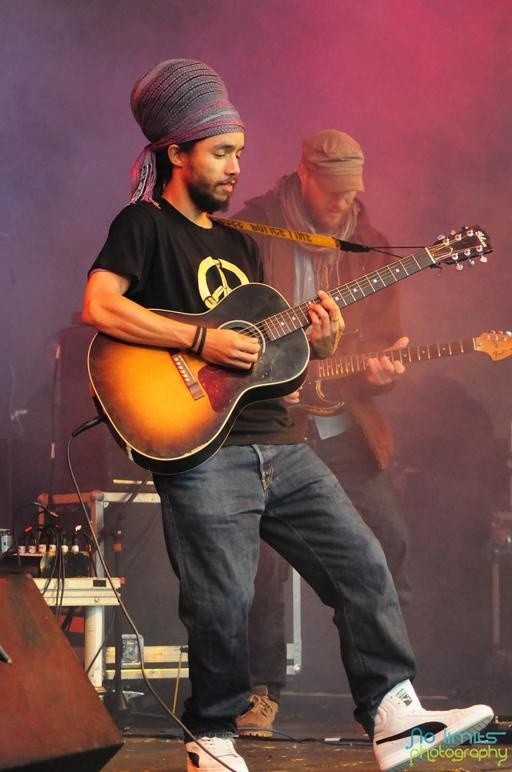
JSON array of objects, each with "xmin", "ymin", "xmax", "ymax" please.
[{"xmin": 332, "ymin": 318, "xmax": 339, "ymax": 322}]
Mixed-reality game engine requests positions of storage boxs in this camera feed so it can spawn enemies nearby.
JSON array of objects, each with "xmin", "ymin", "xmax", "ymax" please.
[{"xmin": 37, "ymin": 491, "xmax": 302, "ymax": 728}]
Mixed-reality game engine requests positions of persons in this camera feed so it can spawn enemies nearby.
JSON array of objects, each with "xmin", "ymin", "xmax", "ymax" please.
[
  {"xmin": 77, "ymin": 59, "xmax": 493, "ymax": 771},
  {"xmin": 228, "ymin": 129, "xmax": 414, "ymax": 739}
]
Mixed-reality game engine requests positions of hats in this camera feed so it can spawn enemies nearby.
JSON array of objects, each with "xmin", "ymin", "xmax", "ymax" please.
[{"xmin": 299, "ymin": 126, "xmax": 369, "ymax": 198}]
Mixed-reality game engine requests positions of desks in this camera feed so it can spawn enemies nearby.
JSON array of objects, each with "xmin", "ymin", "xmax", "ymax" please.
[{"xmin": 33, "ymin": 577, "xmax": 120, "ymax": 702}]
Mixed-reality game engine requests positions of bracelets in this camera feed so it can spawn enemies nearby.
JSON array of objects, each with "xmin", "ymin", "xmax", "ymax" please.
[
  {"xmin": 188, "ymin": 325, "xmax": 201, "ymax": 351},
  {"xmin": 195, "ymin": 327, "xmax": 208, "ymax": 355}
]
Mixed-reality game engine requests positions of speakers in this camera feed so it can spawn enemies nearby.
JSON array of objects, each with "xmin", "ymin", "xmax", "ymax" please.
[{"xmin": 0, "ymin": 573, "xmax": 124, "ymax": 772}]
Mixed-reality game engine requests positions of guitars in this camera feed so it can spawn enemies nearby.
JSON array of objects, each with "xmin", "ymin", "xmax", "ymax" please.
[
  {"xmin": 87, "ymin": 226, "xmax": 493, "ymax": 476},
  {"xmin": 287, "ymin": 329, "xmax": 512, "ymax": 415}
]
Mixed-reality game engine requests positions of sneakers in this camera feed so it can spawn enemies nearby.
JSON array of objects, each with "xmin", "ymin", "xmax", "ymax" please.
[
  {"xmin": 368, "ymin": 679, "xmax": 496, "ymax": 772},
  {"xmin": 232, "ymin": 684, "xmax": 280, "ymax": 739},
  {"xmin": 182, "ymin": 737, "xmax": 251, "ymax": 772}
]
